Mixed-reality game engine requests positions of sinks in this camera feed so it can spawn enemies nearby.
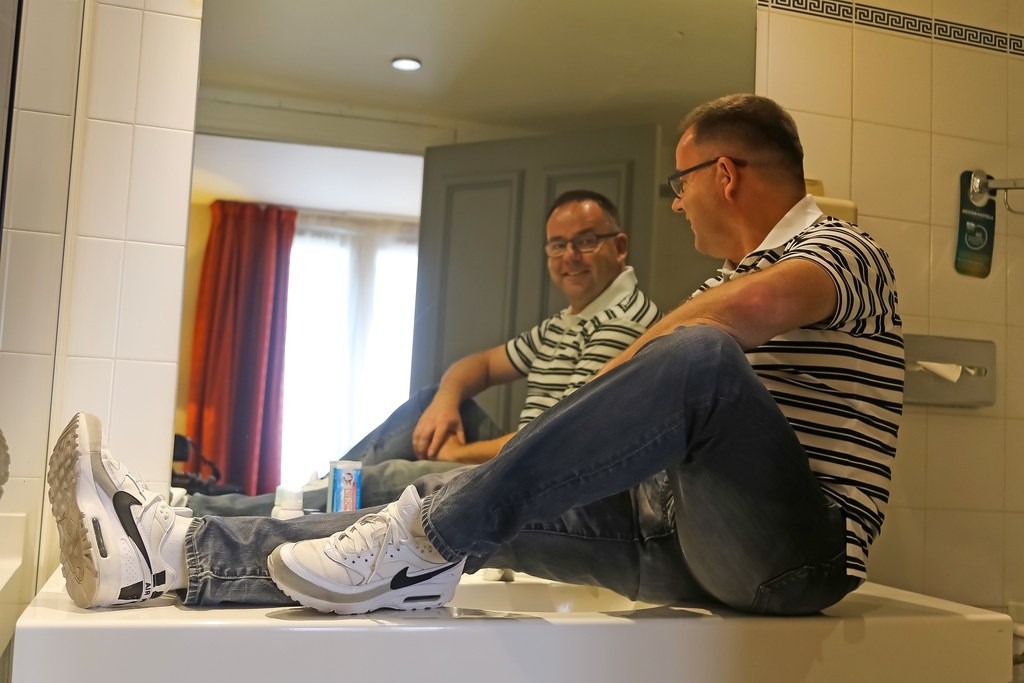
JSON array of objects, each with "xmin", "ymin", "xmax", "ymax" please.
[{"xmin": 410, "ymin": 577, "xmax": 672, "ymax": 614}]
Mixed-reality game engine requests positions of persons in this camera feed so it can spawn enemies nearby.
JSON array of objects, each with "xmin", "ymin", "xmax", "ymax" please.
[
  {"xmin": 169, "ymin": 187, "xmax": 678, "ymax": 517},
  {"xmin": 45, "ymin": 92, "xmax": 907, "ymax": 618}
]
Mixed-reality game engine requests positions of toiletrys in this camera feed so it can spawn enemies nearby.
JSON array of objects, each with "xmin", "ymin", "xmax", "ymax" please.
[
  {"xmin": 278, "ymin": 483, "xmax": 305, "ymax": 520},
  {"xmin": 270, "ymin": 485, "xmax": 284, "ymax": 519},
  {"xmin": 327, "ymin": 461, "xmax": 365, "ymax": 512}
]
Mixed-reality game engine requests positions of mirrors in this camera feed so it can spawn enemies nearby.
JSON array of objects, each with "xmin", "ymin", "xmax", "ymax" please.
[
  {"xmin": 166, "ymin": 0, "xmax": 762, "ymax": 519},
  {"xmin": 0, "ymin": 0, "xmax": 99, "ymax": 680}
]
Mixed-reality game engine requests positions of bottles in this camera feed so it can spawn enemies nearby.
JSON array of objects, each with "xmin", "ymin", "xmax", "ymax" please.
[
  {"xmin": 279, "ymin": 489, "xmax": 304, "ymax": 519},
  {"xmin": 271, "ymin": 486, "xmax": 289, "ymax": 519}
]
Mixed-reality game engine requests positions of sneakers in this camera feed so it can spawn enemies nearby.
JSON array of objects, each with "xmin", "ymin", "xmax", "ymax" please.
[
  {"xmin": 267, "ymin": 484, "xmax": 468, "ymax": 614},
  {"xmin": 47, "ymin": 413, "xmax": 193, "ymax": 609},
  {"xmin": 169, "ymin": 487, "xmax": 188, "ymax": 507},
  {"xmin": 300, "ymin": 472, "xmax": 330, "ymax": 492}
]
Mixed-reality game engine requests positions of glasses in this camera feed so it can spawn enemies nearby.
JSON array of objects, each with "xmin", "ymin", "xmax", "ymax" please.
[
  {"xmin": 544, "ymin": 233, "xmax": 620, "ymax": 257},
  {"xmin": 667, "ymin": 157, "xmax": 747, "ymax": 199}
]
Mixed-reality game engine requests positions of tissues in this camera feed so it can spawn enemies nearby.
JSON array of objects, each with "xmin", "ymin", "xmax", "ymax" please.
[{"xmin": 903, "ymin": 333, "xmax": 997, "ymax": 409}]
